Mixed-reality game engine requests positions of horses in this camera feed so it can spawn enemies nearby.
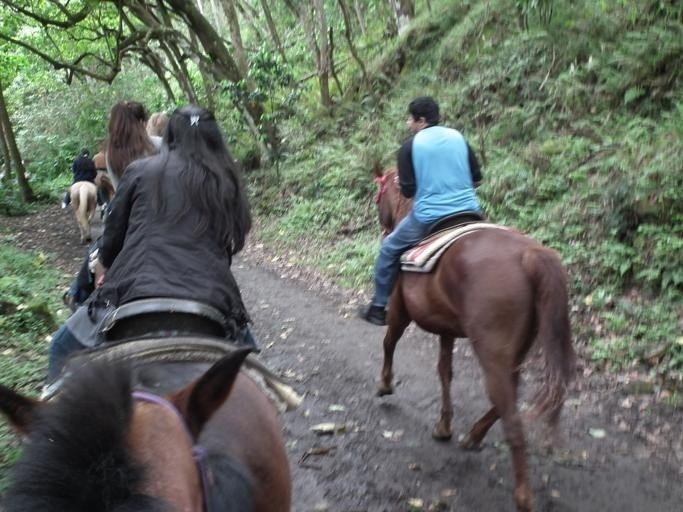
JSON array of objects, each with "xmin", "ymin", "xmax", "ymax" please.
[
  {"xmin": 0, "ymin": 344, "xmax": 259, "ymax": 512},
  {"xmin": 71, "ymin": 180, "xmax": 97, "ymax": 243},
  {"xmin": 48, "ymin": 322, "xmax": 294, "ymax": 512},
  {"xmin": 94, "ymin": 175, "xmax": 116, "ymax": 223},
  {"xmin": 368, "ymin": 157, "xmax": 575, "ymax": 512}
]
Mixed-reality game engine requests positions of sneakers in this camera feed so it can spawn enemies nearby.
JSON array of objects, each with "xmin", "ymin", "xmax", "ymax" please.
[{"xmin": 354, "ymin": 303, "xmax": 388, "ymax": 328}]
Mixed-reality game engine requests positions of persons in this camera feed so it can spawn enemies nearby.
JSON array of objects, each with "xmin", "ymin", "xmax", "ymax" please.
[
  {"xmin": 41, "ymin": 106, "xmax": 259, "ymax": 389},
  {"xmin": 91, "ymin": 144, "xmax": 108, "ymax": 177},
  {"xmin": 358, "ymin": 97, "xmax": 484, "ymax": 327},
  {"xmin": 146, "ymin": 111, "xmax": 169, "ymax": 137},
  {"xmin": 63, "ymin": 98, "xmax": 166, "ymax": 312},
  {"xmin": 62, "ymin": 147, "xmax": 107, "ymax": 211}
]
